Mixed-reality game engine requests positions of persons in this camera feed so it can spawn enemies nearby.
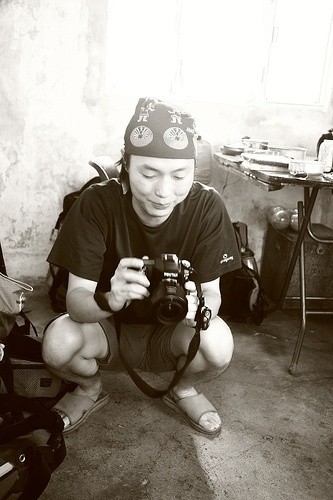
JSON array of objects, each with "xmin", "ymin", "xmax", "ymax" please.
[{"xmin": 40, "ymin": 98, "xmax": 244, "ymax": 437}]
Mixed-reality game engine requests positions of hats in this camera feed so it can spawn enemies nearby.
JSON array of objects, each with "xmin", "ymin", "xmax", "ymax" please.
[{"xmin": 124, "ymin": 95, "xmax": 199, "ymax": 159}]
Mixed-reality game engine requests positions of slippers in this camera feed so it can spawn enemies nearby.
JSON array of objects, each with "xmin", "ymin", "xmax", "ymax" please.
[
  {"xmin": 50, "ymin": 388, "xmax": 110, "ymax": 435},
  {"xmin": 162, "ymin": 392, "xmax": 222, "ymax": 435}
]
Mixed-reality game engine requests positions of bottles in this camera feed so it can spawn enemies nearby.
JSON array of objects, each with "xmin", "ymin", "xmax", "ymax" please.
[{"xmin": 265, "ymin": 205, "xmax": 302, "ymax": 232}]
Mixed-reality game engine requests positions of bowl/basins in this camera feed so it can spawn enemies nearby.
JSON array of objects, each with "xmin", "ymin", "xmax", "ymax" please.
[{"xmin": 278, "ymin": 147, "xmax": 306, "ymax": 161}]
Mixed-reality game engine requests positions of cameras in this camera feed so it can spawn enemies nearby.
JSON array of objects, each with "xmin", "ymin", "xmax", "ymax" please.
[{"xmin": 129, "ymin": 253, "xmax": 191, "ymax": 327}]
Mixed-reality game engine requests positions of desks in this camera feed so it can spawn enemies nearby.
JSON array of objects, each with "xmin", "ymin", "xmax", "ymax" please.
[{"xmin": 213, "ymin": 150, "xmax": 332, "ymax": 374}]
